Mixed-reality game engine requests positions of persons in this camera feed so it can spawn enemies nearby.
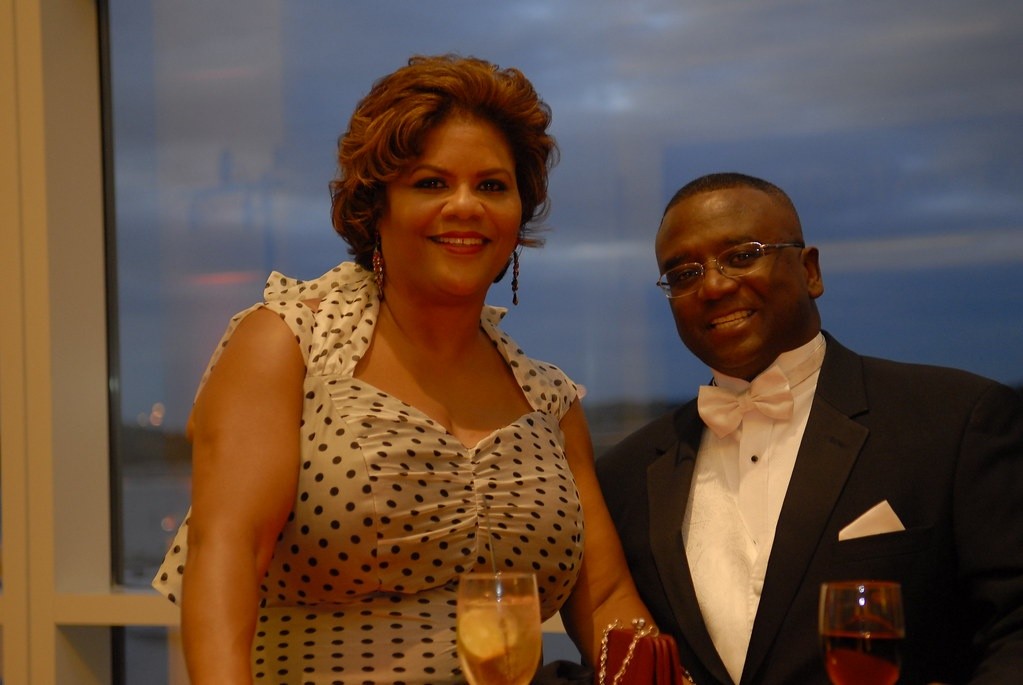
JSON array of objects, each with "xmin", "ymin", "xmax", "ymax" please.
[
  {"xmin": 146, "ymin": 49, "xmax": 660, "ymax": 685},
  {"xmin": 556, "ymin": 172, "xmax": 1023, "ymax": 684}
]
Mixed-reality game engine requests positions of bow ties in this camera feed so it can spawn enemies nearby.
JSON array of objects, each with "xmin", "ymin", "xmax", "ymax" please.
[{"xmin": 698, "ymin": 335, "xmax": 827, "ymax": 439}]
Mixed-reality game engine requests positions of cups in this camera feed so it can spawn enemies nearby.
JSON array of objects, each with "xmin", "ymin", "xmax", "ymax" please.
[
  {"xmin": 455, "ymin": 572, "xmax": 542, "ymax": 685},
  {"xmin": 819, "ymin": 581, "xmax": 906, "ymax": 685}
]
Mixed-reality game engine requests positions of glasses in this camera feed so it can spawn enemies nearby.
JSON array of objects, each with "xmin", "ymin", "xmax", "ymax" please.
[{"xmin": 656, "ymin": 241, "xmax": 805, "ymax": 298}]
